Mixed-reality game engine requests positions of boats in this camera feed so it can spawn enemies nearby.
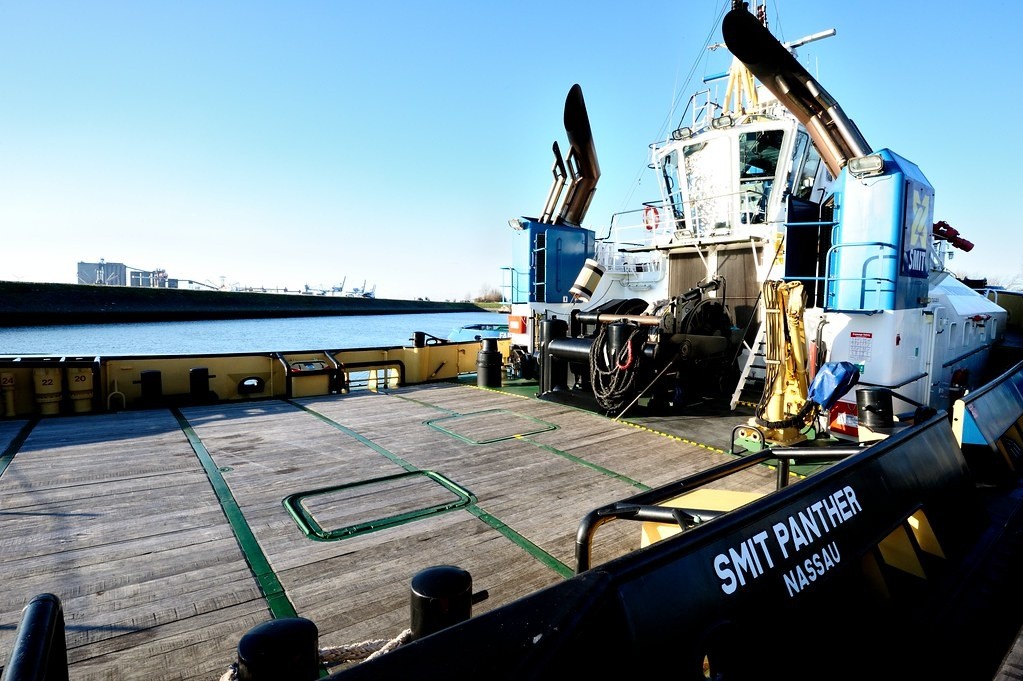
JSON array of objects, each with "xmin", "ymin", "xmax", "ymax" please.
[{"xmin": 1, "ymin": 1, "xmax": 1023, "ymax": 681}]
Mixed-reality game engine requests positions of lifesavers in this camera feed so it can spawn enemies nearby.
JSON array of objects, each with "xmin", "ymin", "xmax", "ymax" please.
[{"xmin": 643, "ymin": 205, "xmax": 660, "ymax": 232}]
